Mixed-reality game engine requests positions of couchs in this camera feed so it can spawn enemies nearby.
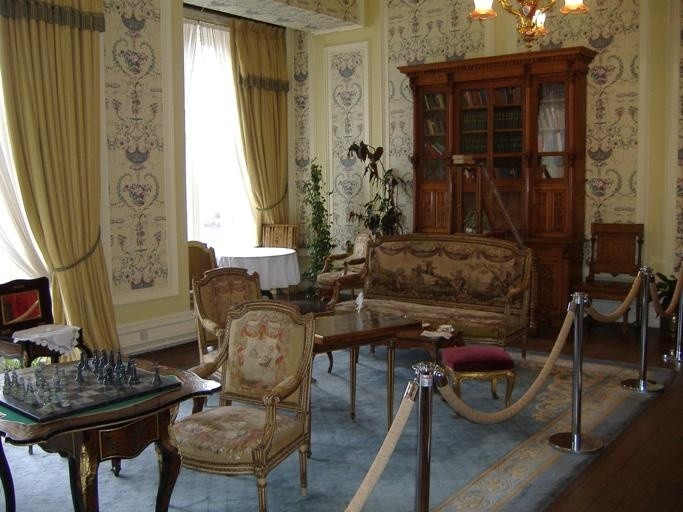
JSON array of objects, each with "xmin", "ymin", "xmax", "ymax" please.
[{"xmin": 327, "ymin": 234, "xmax": 537, "ymax": 358}]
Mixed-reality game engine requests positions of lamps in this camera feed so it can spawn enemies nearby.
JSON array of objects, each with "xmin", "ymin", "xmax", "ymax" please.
[{"xmin": 470, "ymin": 0, "xmax": 589, "ymax": 47}]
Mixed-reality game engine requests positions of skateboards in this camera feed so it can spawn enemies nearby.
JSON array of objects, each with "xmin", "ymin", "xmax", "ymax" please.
[{"xmin": 0, "ymin": 347, "xmax": 181, "ymax": 423}]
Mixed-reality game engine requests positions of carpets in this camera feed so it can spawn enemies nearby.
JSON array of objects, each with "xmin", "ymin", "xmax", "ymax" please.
[{"xmin": 0, "ymin": 344, "xmax": 677, "ymax": 512}]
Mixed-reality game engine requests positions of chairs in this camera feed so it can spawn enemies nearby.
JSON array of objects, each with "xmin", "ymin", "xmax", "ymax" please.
[
  {"xmin": 158, "ymin": 300, "xmax": 317, "ymax": 512},
  {"xmin": 317, "ymin": 233, "xmax": 373, "ymax": 301},
  {"xmin": 573, "ymin": 222, "xmax": 645, "ymax": 335},
  {"xmin": 187, "ymin": 241, "xmax": 220, "ymax": 290},
  {"xmin": 192, "ymin": 266, "xmax": 263, "ymax": 406},
  {"xmin": 0, "ymin": 276, "xmax": 93, "ymax": 369}
]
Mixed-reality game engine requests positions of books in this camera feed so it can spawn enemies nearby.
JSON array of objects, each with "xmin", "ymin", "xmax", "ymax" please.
[{"xmin": 423, "ymin": 83, "xmax": 566, "ymax": 181}]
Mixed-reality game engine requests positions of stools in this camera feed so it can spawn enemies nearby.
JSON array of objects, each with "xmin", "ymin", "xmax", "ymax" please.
[{"xmin": 438, "ymin": 347, "xmax": 515, "ymax": 417}]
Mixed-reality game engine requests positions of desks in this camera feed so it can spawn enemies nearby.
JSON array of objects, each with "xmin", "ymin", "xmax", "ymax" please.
[
  {"xmin": 311, "ymin": 312, "xmax": 464, "ymax": 430},
  {"xmin": 0, "ymin": 356, "xmax": 221, "ymax": 512},
  {"xmin": 217, "ymin": 247, "xmax": 301, "ymax": 300}
]
